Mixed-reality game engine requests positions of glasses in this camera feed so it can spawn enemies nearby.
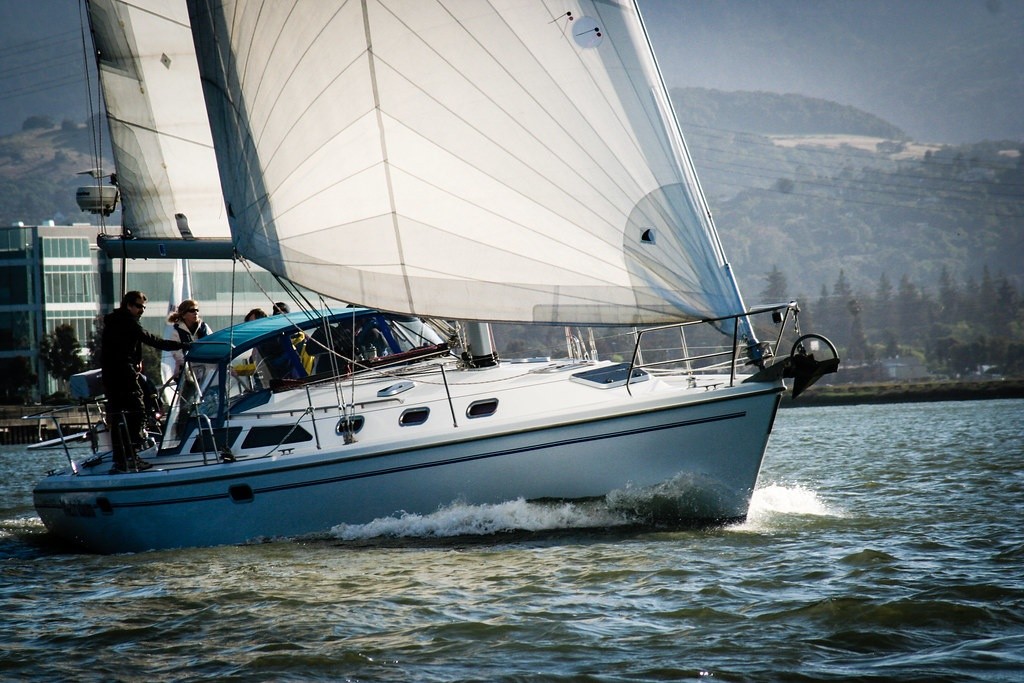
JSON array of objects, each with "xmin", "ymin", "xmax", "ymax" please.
[
  {"xmin": 133, "ymin": 303, "xmax": 146, "ymax": 309},
  {"xmin": 188, "ymin": 308, "xmax": 199, "ymax": 312}
]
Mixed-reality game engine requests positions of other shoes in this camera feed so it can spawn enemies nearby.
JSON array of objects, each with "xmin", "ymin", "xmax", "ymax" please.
[{"xmin": 128, "ymin": 455, "xmax": 152, "ymax": 469}]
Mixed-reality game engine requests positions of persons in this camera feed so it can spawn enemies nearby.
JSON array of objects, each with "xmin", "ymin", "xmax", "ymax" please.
[
  {"xmin": 305, "ymin": 304, "xmax": 389, "ymax": 374},
  {"xmin": 272, "ymin": 302, "xmax": 314, "ymax": 375},
  {"xmin": 230, "ymin": 308, "xmax": 272, "ymax": 388},
  {"xmin": 132, "ymin": 361, "xmax": 161, "ymax": 419},
  {"xmin": 168, "ymin": 300, "xmax": 214, "ymax": 407},
  {"xmin": 99, "ymin": 290, "xmax": 192, "ymax": 477}
]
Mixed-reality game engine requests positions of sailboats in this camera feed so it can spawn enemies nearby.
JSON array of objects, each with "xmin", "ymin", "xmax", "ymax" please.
[{"xmin": 21, "ymin": 0, "xmax": 845, "ymax": 558}]
